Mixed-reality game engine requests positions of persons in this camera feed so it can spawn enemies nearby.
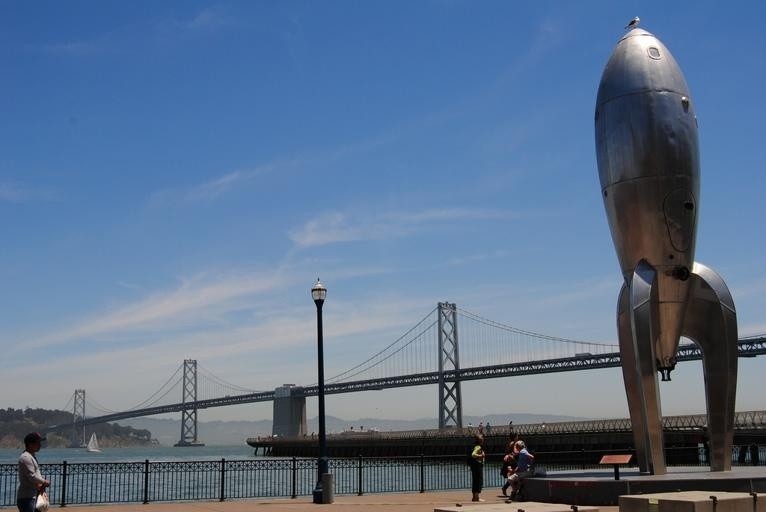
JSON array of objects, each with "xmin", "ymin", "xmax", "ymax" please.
[
  {"xmin": 509, "ymin": 421, "xmax": 513, "ymax": 433},
  {"xmin": 16, "ymin": 431, "xmax": 50, "ymax": 512},
  {"xmin": 501, "ymin": 432, "xmax": 534, "ymax": 503},
  {"xmin": 467, "ymin": 433, "xmax": 486, "ymax": 502},
  {"xmin": 486, "ymin": 422, "xmax": 490, "ymax": 435},
  {"xmin": 468, "ymin": 422, "xmax": 473, "ymax": 435},
  {"xmin": 479, "ymin": 422, "xmax": 484, "ymax": 434}
]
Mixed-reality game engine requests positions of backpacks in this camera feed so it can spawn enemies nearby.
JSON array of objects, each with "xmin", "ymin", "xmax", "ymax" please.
[{"xmin": 466, "ymin": 447, "xmax": 475, "ymax": 464}]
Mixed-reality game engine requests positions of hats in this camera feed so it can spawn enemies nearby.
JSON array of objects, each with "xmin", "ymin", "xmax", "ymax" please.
[{"xmin": 24, "ymin": 433, "xmax": 45, "ymax": 442}]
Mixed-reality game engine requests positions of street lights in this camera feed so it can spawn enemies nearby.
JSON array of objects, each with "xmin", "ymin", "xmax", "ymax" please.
[{"xmin": 311, "ymin": 277, "xmax": 328, "ymax": 504}]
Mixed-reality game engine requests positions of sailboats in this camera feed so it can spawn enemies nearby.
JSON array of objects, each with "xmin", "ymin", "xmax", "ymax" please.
[{"xmin": 87, "ymin": 432, "xmax": 104, "ymax": 452}]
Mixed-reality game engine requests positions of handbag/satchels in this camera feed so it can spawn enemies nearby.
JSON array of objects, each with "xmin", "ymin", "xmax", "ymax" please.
[{"xmin": 35, "ymin": 489, "xmax": 49, "ymax": 512}]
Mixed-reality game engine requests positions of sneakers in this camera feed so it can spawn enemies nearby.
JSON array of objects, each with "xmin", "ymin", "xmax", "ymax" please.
[
  {"xmin": 502, "ymin": 487, "xmax": 515, "ymax": 500},
  {"xmin": 472, "ymin": 499, "xmax": 484, "ymax": 501}
]
